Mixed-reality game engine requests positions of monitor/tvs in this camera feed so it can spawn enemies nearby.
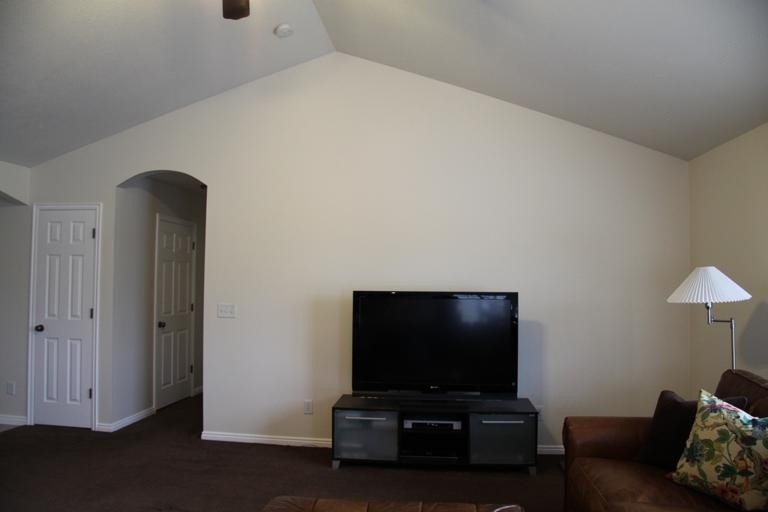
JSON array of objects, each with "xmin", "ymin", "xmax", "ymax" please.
[{"xmin": 352, "ymin": 291, "xmax": 518, "ymax": 401}]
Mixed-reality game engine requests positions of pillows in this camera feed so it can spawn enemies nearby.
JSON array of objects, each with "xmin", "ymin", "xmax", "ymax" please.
[{"xmin": 645, "ymin": 386, "xmax": 768, "ymax": 509}]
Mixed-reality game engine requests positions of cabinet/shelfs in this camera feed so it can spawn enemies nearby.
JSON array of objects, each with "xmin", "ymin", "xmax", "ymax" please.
[{"xmin": 330, "ymin": 393, "xmax": 539, "ymax": 478}]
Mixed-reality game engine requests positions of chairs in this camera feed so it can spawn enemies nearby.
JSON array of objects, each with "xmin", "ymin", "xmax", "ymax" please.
[{"xmin": 559, "ymin": 367, "xmax": 768, "ymax": 511}]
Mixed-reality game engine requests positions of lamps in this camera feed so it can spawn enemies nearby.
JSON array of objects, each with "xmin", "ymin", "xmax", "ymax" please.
[{"xmin": 665, "ymin": 263, "xmax": 754, "ymax": 371}]
